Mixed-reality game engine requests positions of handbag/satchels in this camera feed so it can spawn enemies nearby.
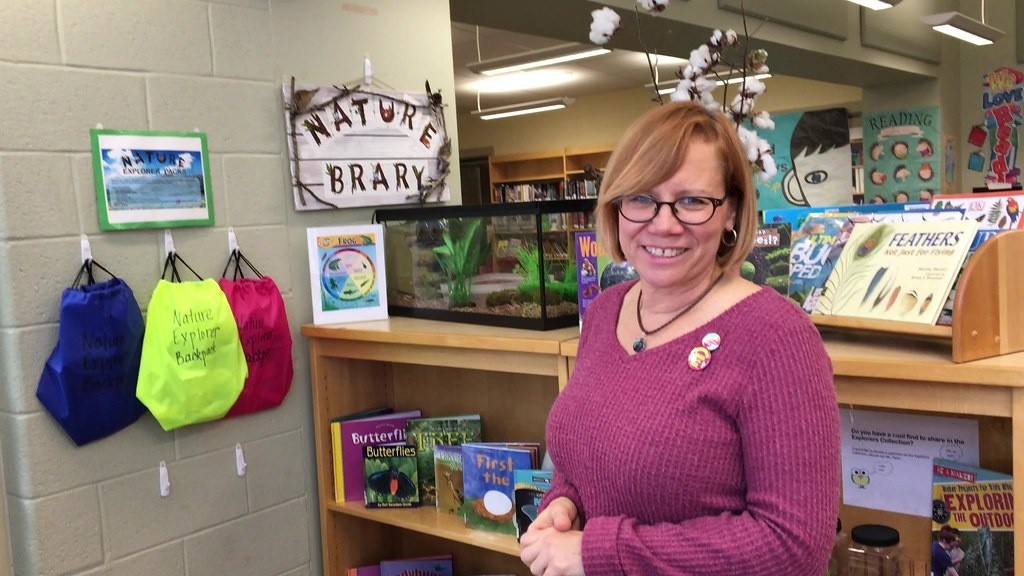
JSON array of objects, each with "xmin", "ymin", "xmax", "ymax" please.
[
  {"xmin": 29, "ymin": 260, "xmax": 147, "ymax": 451},
  {"xmin": 217, "ymin": 249, "xmax": 293, "ymax": 420},
  {"xmin": 134, "ymin": 250, "xmax": 247, "ymax": 430}
]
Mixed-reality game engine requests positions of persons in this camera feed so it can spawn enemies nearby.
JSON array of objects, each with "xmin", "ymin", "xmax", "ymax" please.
[{"xmin": 519, "ymin": 101, "xmax": 841, "ymax": 575}]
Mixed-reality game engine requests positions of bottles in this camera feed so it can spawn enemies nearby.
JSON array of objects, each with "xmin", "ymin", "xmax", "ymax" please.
[
  {"xmin": 839, "ymin": 521, "xmax": 903, "ymax": 575},
  {"xmin": 825, "ymin": 515, "xmax": 851, "ymax": 576}
]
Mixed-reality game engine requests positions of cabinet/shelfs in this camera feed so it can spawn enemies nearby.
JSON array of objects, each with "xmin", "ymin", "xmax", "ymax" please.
[
  {"xmin": 301, "ymin": 317, "xmax": 579, "ymax": 576},
  {"xmin": 806, "ymin": 229, "xmax": 1023, "ymax": 576}
]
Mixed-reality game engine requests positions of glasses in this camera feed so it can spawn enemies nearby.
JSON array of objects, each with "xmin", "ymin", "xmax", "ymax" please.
[{"xmin": 612, "ymin": 193, "xmax": 731, "ymax": 226}]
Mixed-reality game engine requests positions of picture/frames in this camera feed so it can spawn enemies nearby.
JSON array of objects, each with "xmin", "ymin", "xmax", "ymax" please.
[
  {"xmin": 282, "ymin": 81, "xmax": 451, "ymax": 211},
  {"xmin": 91, "ymin": 129, "xmax": 215, "ymax": 231}
]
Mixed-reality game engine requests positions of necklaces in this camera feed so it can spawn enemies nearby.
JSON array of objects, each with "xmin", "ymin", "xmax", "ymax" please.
[{"xmin": 631, "ymin": 270, "xmax": 728, "ymax": 355}]
